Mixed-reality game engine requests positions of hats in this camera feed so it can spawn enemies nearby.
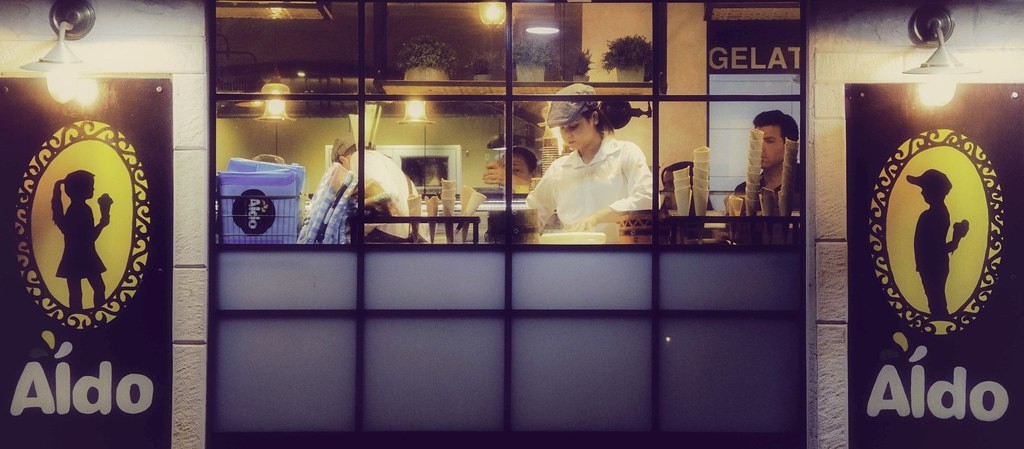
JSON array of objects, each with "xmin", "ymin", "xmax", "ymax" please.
[
  {"xmin": 547, "ymin": 83, "xmax": 599, "ymax": 128},
  {"xmin": 331, "ymin": 137, "xmax": 355, "ymax": 164}
]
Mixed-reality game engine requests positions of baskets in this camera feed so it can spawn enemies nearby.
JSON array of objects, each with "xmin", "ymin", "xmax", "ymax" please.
[{"xmin": 216, "ymin": 195, "xmax": 298, "ymax": 244}]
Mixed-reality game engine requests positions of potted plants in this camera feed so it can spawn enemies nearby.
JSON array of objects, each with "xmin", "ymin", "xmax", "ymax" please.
[
  {"xmin": 571, "ymin": 46, "xmax": 594, "ymax": 81},
  {"xmin": 600, "ymin": 34, "xmax": 651, "ymax": 82},
  {"xmin": 502, "ymin": 34, "xmax": 563, "ymax": 81},
  {"xmin": 396, "ymin": 35, "xmax": 459, "ymax": 81}
]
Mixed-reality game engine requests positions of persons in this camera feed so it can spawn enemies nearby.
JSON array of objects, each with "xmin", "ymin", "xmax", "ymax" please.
[
  {"xmin": 482, "ymin": 146, "xmax": 538, "ymax": 192},
  {"xmin": 525, "ymin": 83, "xmax": 660, "ymax": 244},
  {"xmin": 735, "ymin": 110, "xmax": 800, "ymax": 216},
  {"xmin": 331, "ymin": 137, "xmax": 418, "ymax": 243}
]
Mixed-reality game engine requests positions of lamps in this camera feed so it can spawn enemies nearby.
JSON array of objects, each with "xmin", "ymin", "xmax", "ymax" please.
[
  {"xmin": 253, "ymin": 61, "xmax": 295, "ymax": 122},
  {"xmin": 478, "ymin": 2, "xmax": 507, "ymax": 33},
  {"xmin": 902, "ymin": 5, "xmax": 982, "ymax": 105},
  {"xmin": 396, "ymin": 101, "xmax": 436, "ymax": 124},
  {"xmin": 40, "ymin": 0, "xmax": 96, "ymax": 103}
]
